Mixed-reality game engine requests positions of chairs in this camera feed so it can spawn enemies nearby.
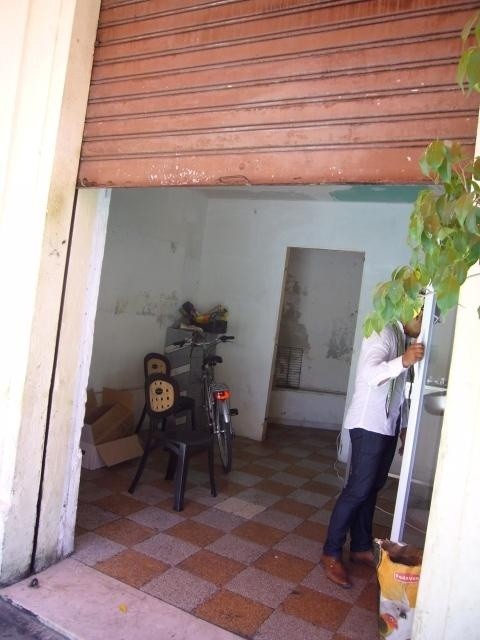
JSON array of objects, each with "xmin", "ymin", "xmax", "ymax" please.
[
  {"xmin": 135, "ymin": 353, "xmax": 195, "ymax": 432},
  {"xmin": 128, "ymin": 373, "xmax": 217, "ymax": 512}
]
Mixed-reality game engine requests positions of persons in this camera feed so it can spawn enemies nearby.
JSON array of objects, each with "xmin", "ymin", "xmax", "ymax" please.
[{"xmin": 321, "ymin": 298, "xmax": 442, "ymax": 587}]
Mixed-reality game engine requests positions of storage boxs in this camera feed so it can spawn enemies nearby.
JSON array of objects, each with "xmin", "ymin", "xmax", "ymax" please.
[{"xmin": 81, "ymin": 389, "xmax": 139, "ymax": 470}]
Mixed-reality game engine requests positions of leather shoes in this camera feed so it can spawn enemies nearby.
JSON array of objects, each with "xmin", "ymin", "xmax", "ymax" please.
[
  {"xmin": 321, "ymin": 554, "xmax": 350, "ymax": 585},
  {"xmin": 350, "ymin": 550, "xmax": 377, "ymax": 567}
]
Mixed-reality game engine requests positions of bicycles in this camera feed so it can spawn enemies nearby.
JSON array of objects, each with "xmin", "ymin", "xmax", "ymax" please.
[{"xmin": 173, "ymin": 336, "xmax": 238, "ymax": 473}]
